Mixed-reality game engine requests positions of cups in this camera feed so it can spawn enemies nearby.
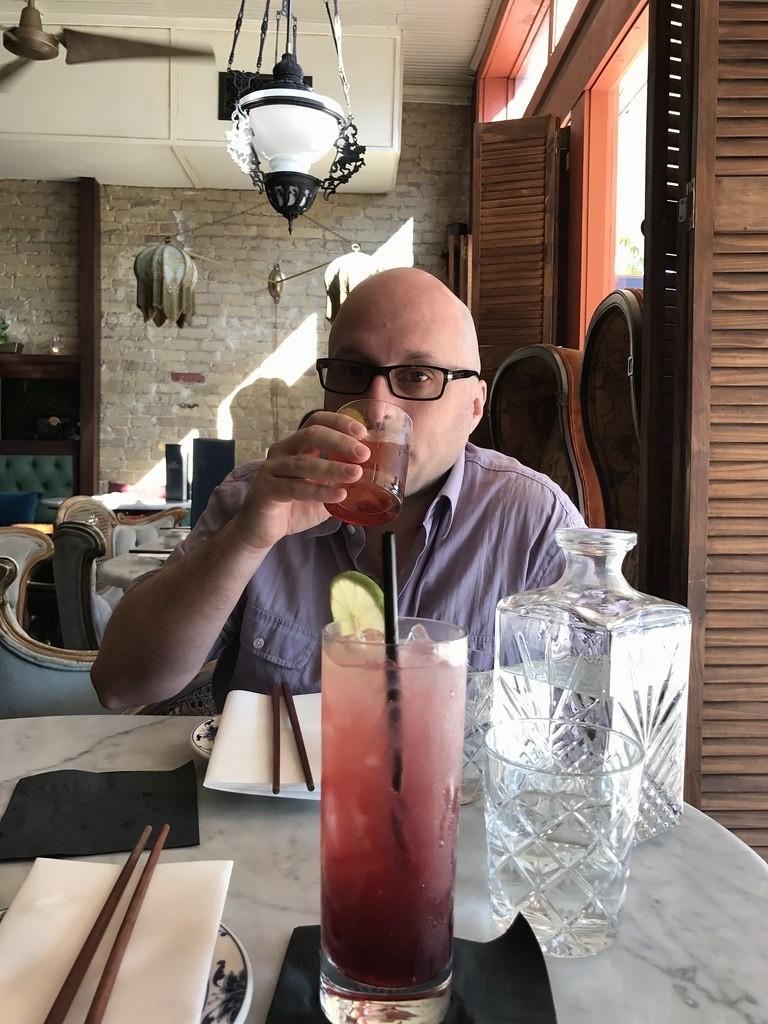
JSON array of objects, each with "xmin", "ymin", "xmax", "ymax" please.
[
  {"xmin": 459, "ymin": 648, "xmax": 499, "ymax": 804},
  {"xmin": 484, "ymin": 719, "xmax": 646, "ymax": 960},
  {"xmin": 323, "ymin": 399, "xmax": 413, "ymax": 527},
  {"xmin": 320, "ymin": 619, "xmax": 467, "ymax": 1024}
]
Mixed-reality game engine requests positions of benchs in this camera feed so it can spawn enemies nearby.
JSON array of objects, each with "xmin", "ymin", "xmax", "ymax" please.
[{"xmin": 0, "ymin": 440, "xmax": 80, "ymax": 524}]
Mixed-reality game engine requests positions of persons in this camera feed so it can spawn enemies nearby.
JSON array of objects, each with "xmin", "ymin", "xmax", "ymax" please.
[{"xmin": 89, "ymin": 268, "xmax": 590, "ymax": 710}]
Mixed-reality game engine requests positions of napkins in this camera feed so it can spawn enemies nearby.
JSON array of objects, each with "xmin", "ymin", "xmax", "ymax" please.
[
  {"xmin": 0, "ymin": 759, "xmax": 201, "ymax": 866},
  {"xmin": 265, "ymin": 911, "xmax": 557, "ymax": 1024},
  {"xmin": 0, "ymin": 858, "xmax": 233, "ymax": 1024},
  {"xmin": 203, "ymin": 690, "xmax": 322, "ymax": 801}
]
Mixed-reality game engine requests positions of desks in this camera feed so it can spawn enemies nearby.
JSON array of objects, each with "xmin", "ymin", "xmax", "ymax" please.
[
  {"xmin": 41, "ymin": 498, "xmax": 192, "ymax": 528},
  {"xmin": 0, "ymin": 714, "xmax": 768, "ymax": 1024},
  {"xmin": 97, "ymin": 553, "xmax": 163, "ymax": 595}
]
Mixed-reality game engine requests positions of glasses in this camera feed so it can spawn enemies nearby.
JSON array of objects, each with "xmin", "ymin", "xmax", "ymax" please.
[{"xmin": 316, "ymin": 358, "xmax": 480, "ymax": 401}]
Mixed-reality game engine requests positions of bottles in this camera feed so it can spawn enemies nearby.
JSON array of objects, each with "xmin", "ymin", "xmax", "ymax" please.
[{"xmin": 493, "ymin": 527, "xmax": 692, "ymax": 847}]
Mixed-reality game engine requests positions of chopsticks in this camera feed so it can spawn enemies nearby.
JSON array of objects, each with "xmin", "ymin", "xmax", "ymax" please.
[
  {"xmin": 45, "ymin": 825, "xmax": 171, "ymax": 1024},
  {"xmin": 272, "ymin": 680, "xmax": 314, "ymax": 794}
]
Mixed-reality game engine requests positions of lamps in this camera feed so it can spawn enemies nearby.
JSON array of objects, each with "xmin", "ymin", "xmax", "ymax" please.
[{"xmin": 225, "ymin": 0, "xmax": 366, "ymax": 234}]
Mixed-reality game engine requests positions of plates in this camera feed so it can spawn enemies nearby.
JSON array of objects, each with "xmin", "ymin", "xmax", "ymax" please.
[
  {"xmin": 0, "ymin": 908, "xmax": 254, "ymax": 1024},
  {"xmin": 190, "ymin": 716, "xmax": 220, "ymax": 757}
]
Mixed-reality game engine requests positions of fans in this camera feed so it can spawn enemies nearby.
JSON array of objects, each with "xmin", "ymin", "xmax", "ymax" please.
[{"xmin": 0, "ymin": 0, "xmax": 217, "ymax": 91}]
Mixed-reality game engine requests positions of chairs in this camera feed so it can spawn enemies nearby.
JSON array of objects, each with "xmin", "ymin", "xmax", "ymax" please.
[
  {"xmin": 119, "ymin": 658, "xmax": 218, "ymax": 717},
  {"xmin": 0, "ymin": 526, "xmax": 128, "ymax": 720},
  {"xmin": 52, "ymin": 496, "xmax": 188, "ymax": 651}
]
